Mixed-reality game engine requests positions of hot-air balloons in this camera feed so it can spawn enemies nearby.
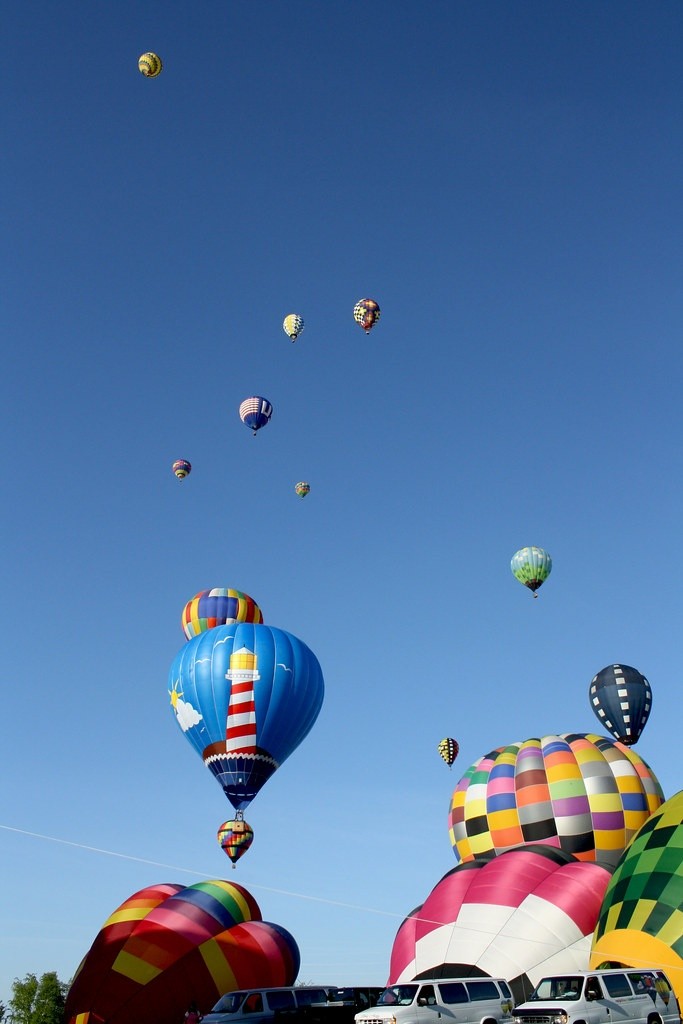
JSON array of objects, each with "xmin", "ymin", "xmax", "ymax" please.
[
  {"xmin": 510, "ymin": 546, "xmax": 553, "ymax": 599},
  {"xmin": 239, "ymin": 396, "xmax": 274, "ymax": 436},
  {"xmin": 640, "ymin": 975, "xmax": 657, "ymax": 1009},
  {"xmin": 138, "ymin": 51, "xmax": 163, "ymax": 79},
  {"xmin": 386, "ymin": 843, "xmax": 614, "ymax": 1012},
  {"xmin": 181, "ymin": 587, "xmax": 265, "ymax": 643},
  {"xmin": 167, "ymin": 623, "xmax": 326, "ymax": 820},
  {"xmin": 589, "ymin": 663, "xmax": 652, "ymax": 750},
  {"xmin": 217, "ymin": 819, "xmax": 254, "ymax": 870},
  {"xmin": 500, "ymin": 1000, "xmax": 509, "ymax": 1016},
  {"xmin": 353, "ymin": 298, "xmax": 381, "ymax": 334},
  {"xmin": 172, "ymin": 459, "xmax": 191, "ymax": 483},
  {"xmin": 295, "ymin": 482, "xmax": 310, "ymax": 500},
  {"xmin": 588, "ymin": 789, "xmax": 683, "ymax": 1024},
  {"xmin": 438, "ymin": 737, "xmax": 460, "ymax": 771},
  {"xmin": 446, "ymin": 733, "xmax": 666, "ymax": 866},
  {"xmin": 60, "ymin": 880, "xmax": 300, "ymax": 1024},
  {"xmin": 283, "ymin": 314, "xmax": 304, "ymax": 343},
  {"xmin": 507, "ymin": 1000, "xmax": 513, "ymax": 1016},
  {"xmin": 653, "ymin": 978, "xmax": 672, "ymax": 1012}
]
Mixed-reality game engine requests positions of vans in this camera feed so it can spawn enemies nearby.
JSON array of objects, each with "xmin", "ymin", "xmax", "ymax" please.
[
  {"xmin": 200, "ymin": 986, "xmax": 339, "ymax": 1024},
  {"xmin": 512, "ymin": 967, "xmax": 681, "ymax": 1024},
  {"xmin": 354, "ymin": 977, "xmax": 517, "ymax": 1024}
]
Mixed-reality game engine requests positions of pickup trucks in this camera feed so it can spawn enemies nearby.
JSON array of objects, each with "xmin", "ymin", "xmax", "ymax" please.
[{"xmin": 273, "ymin": 986, "xmax": 399, "ymax": 1024}]
